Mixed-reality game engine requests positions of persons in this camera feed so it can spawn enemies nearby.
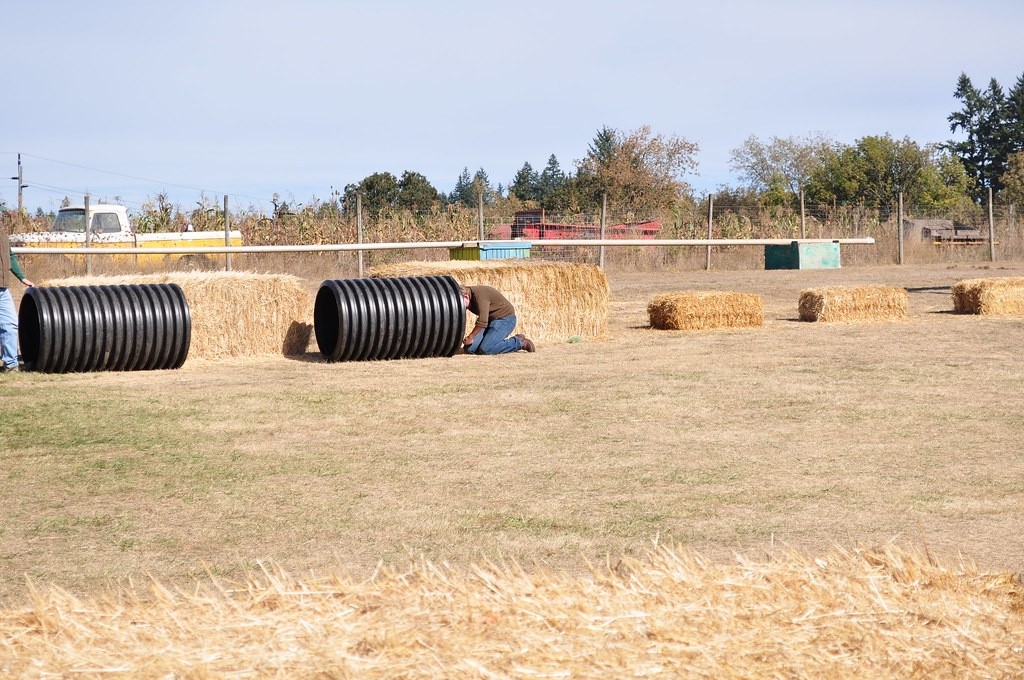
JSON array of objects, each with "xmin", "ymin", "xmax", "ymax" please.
[
  {"xmin": 0, "ymin": 206, "xmax": 35, "ymax": 373},
  {"xmin": 459, "ymin": 285, "xmax": 535, "ymax": 354}
]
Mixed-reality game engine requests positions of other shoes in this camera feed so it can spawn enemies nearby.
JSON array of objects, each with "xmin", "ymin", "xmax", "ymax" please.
[{"xmin": 515, "ymin": 334, "xmax": 536, "ymax": 353}]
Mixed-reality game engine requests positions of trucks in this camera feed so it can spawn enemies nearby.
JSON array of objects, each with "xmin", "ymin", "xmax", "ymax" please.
[
  {"xmin": 483, "ymin": 207, "xmax": 660, "ymax": 240},
  {"xmin": 6, "ymin": 204, "xmax": 244, "ymax": 249}
]
